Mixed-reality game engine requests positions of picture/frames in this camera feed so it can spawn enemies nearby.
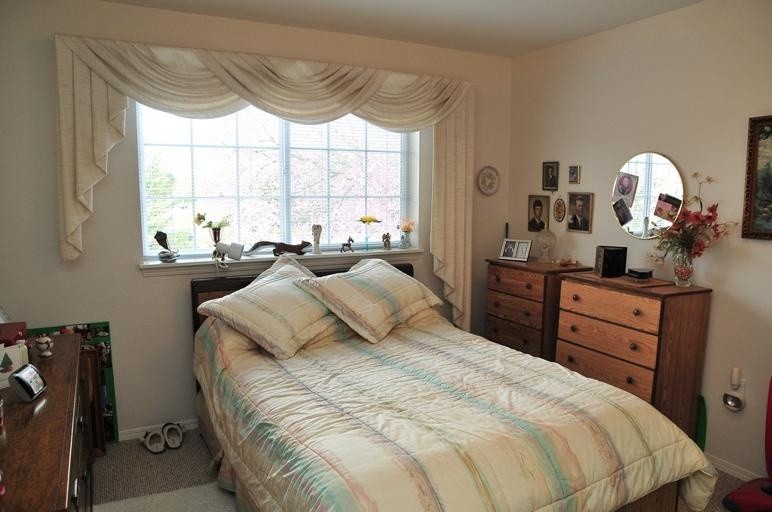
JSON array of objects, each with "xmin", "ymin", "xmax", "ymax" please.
[
  {"xmin": 476, "ymin": 166, "xmax": 500, "ymax": 196},
  {"xmin": 527, "ymin": 162, "xmax": 595, "ymax": 234},
  {"xmin": 741, "ymin": 114, "xmax": 772, "ymax": 239},
  {"xmin": 499, "ymin": 238, "xmax": 533, "ymax": 262}
]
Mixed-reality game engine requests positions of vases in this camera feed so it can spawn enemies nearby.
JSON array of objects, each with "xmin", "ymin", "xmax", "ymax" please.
[
  {"xmin": 400, "ymin": 231, "xmax": 410, "ymax": 248},
  {"xmin": 364, "ymin": 236, "xmax": 370, "ymax": 251},
  {"xmin": 673, "ymin": 255, "xmax": 695, "ymax": 287},
  {"xmin": 212, "ymin": 227, "xmax": 221, "ymax": 257}
]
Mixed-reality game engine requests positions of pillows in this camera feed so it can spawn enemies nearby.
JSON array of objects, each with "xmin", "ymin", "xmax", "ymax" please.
[
  {"xmin": 197, "ymin": 253, "xmax": 337, "ymax": 360},
  {"xmin": 292, "ymin": 257, "xmax": 446, "ymax": 344}
]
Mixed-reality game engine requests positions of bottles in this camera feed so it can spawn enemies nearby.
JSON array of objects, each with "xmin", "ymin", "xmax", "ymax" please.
[{"xmin": 17, "ymin": 340, "xmax": 29, "ymax": 365}]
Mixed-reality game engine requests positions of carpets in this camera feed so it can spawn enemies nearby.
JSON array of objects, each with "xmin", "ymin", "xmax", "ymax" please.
[{"xmin": 89, "ymin": 427, "xmax": 217, "ymax": 505}]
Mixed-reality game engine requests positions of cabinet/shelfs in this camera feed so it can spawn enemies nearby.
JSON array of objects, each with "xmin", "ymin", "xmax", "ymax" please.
[
  {"xmin": 555, "ymin": 269, "xmax": 713, "ymax": 430},
  {"xmin": 482, "ymin": 257, "xmax": 593, "ymax": 360},
  {"xmin": 1, "ymin": 331, "xmax": 93, "ymax": 511}
]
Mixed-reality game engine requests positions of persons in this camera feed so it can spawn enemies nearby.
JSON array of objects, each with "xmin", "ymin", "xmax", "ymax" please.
[
  {"xmin": 569, "ymin": 195, "xmax": 588, "ymax": 229},
  {"xmin": 528, "ymin": 199, "xmax": 545, "ymax": 230},
  {"xmin": 620, "ymin": 176, "xmax": 632, "ymax": 194},
  {"xmin": 505, "ymin": 243, "xmax": 512, "ymax": 256},
  {"xmin": 547, "ymin": 168, "xmax": 555, "ymax": 186}
]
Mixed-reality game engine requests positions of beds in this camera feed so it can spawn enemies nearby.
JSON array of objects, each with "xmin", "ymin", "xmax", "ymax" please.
[{"xmin": 190, "ymin": 265, "xmax": 677, "ymax": 511}]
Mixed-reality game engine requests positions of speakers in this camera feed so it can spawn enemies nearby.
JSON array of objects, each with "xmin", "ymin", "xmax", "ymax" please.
[{"xmin": 594, "ymin": 246, "xmax": 627, "ymax": 277}]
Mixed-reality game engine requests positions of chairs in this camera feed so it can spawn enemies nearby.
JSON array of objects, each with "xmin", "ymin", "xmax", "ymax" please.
[{"xmin": 721, "ymin": 377, "xmax": 772, "ymax": 511}]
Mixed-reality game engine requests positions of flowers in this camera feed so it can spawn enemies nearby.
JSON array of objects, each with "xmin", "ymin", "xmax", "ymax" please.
[
  {"xmin": 398, "ymin": 221, "xmax": 415, "ymax": 232},
  {"xmin": 357, "ymin": 215, "xmax": 382, "ymax": 237},
  {"xmin": 195, "ymin": 211, "xmax": 231, "ymax": 240},
  {"xmin": 646, "ymin": 171, "xmax": 740, "ymax": 280}
]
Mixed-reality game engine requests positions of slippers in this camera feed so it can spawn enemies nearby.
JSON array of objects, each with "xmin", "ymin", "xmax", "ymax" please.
[
  {"xmin": 142, "ymin": 431, "xmax": 165, "ymax": 454},
  {"xmin": 161, "ymin": 422, "xmax": 183, "ymax": 449}
]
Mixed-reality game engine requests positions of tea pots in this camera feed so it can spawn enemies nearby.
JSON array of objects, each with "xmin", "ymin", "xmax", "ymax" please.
[{"xmin": 34, "ymin": 332, "xmax": 55, "ymax": 357}]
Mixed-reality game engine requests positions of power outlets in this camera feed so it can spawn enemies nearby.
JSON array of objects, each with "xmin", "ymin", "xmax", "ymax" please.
[{"xmin": 728, "ymin": 379, "xmax": 747, "ymax": 399}]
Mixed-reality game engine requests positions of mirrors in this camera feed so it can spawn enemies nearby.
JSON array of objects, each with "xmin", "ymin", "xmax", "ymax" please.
[{"xmin": 610, "ymin": 151, "xmax": 684, "ymax": 240}]
